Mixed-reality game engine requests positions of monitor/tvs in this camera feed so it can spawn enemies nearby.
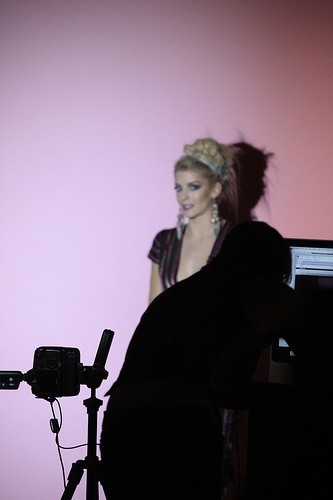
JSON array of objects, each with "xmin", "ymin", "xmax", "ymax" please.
[
  {"xmin": 283, "ymin": 238, "xmax": 333, "ymax": 290},
  {"xmin": 295, "ymin": 275, "xmax": 333, "ymax": 292}
]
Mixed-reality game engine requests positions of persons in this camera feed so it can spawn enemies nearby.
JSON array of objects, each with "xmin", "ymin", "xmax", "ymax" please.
[
  {"xmin": 148, "ymin": 139, "xmax": 238, "ymax": 500},
  {"xmin": 98, "ymin": 221, "xmax": 293, "ymax": 500}
]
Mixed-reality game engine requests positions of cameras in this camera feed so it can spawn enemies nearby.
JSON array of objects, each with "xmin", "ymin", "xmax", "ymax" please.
[{"xmin": 0, "ymin": 346, "xmax": 84, "ymax": 399}]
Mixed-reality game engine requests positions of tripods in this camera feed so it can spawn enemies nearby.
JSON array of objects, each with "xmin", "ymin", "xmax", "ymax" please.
[{"xmin": 60, "ymin": 329, "xmax": 116, "ymax": 500}]
